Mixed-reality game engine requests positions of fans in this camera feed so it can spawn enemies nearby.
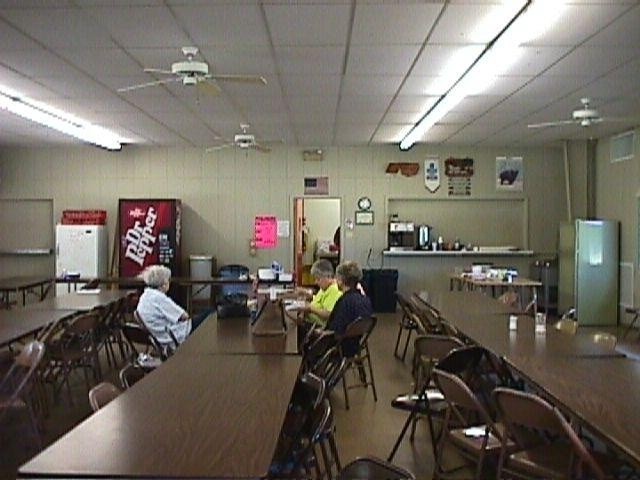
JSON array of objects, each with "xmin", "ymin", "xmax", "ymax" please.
[
  {"xmin": 206, "ymin": 123, "xmax": 284, "ymax": 154},
  {"xmin": 116, "ymin": 46, "xmax": 268, "ymax": 96},
  {"xmin": 528, "ymin": 98, "xmax": 640, "ymax": 140}
]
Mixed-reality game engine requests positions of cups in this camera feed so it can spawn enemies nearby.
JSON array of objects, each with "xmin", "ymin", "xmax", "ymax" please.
[
  {"xmin": 535, "ymin": 312, "xmax": 546, "ymax": 333},
  {"xmin": 432, "ymin": 242, "xmax": 437, "ymax": 250},
  {"xmin": 473, "ymin": 265, "xmax": 482, "ymax": 276}
]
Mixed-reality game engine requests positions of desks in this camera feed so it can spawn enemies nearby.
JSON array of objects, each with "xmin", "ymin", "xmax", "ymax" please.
[{"xmin": 412, "ymin": 290, "xmax": 640, "ymax": 470}]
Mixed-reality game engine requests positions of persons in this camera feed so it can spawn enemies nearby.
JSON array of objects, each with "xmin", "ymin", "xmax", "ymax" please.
[
  {"xmin": 136, "ymin": 264, "xmax": 214, "ymax": 358},
  {"xmin": 290, "ymin": 258, "xmax": 344, "ymax": 353},
  {"xmin": 303, "ymin": 261, "xmax": 373, "ymax": 371}
]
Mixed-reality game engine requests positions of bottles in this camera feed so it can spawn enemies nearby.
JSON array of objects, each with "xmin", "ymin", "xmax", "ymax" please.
[{"xmin": 438, "ymin": 236, "xmax": 460, "ymax": 250}]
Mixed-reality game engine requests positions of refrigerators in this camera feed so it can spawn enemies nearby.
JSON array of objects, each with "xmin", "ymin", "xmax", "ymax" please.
[
  {"xmin": 55, "ymin": 224, "xmax": 107, "ymax": 294},
  {"xmin": 558, "ymin": 219, "xmax": 618, "ymax": 328}
]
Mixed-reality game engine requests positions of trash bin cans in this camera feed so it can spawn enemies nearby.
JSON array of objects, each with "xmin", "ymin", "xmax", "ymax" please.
[{"xmin": 362, "ymin": 268, "xmax": 398, "ymax": 313}]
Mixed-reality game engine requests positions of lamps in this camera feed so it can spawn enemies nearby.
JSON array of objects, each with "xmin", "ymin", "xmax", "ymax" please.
[
  {"xmin": 400, "ymin": 0, "xmax": 563, "ymax": 151},
  {"xmin": 0, "ymin": 93, "xmax": 121, "ymax": 151}
]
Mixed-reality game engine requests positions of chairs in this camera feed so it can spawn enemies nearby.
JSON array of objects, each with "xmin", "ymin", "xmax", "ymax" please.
[
  {"xmin": 395, "ymin": 273, "xmax": 604, "ymax": 480},
  {"xmin": 1, "ymin": 272, "xmax": 416, "ymax": 480}
]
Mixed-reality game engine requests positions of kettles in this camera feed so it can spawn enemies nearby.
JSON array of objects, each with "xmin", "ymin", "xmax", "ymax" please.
[{"xmin": 415, "ymin": 223, "xmax": 432, "ymax": 251}]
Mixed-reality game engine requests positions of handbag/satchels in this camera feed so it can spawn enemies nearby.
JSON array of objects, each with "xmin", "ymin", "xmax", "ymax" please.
[
  {"xmin": 251, "ymin": 294, "xmax": 287, "ymax": 335},
  {"xmin": 217, "ymin": 293, "xmax": 257, "ymax": 319}
]
